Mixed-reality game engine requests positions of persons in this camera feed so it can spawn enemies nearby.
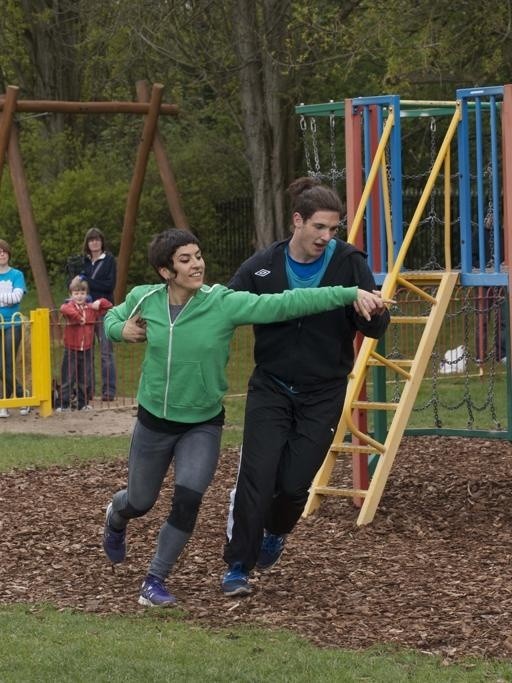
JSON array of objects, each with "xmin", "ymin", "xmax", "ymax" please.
[
  {"xmin": 102, "ymin": 226, "xmax": 398, "ymax": 607},
  {"xmin": 0, "ymin": 239, "xmax": 30, "ymax": 418},
  {"xmin": 472, "ymin": 209, "xmax": 507, "ymax": 366},
  {"xmin": 56, "ymin": 277, "xmax": 113, "ymax": 413},
  {"xmin": 73, "ymin": 227, "xmax": 117, "ymax": 400},
  {"xmin": 222, "ymin": 175, "xmax": 391, "ymax": 598}
]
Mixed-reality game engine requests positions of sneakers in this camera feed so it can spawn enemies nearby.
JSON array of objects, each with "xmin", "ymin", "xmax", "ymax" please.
[
  {"xmin": 103, "ymin": 502, "xmax": 126, "ymax": 563},
  {"xmin": 140, "ymin": 577, "xmax": 176, "ymax": 607},
  {"xmin": 0, "ymin": 389, "xmax": 29, "ymax": 417},
  {"xmin": 222, "ymin": 562, "xmax": 252, "ymax": 597},
  {"xmin": 57, "ymin": 405, "xmax": 93, "ymax": 412},
  {"xmin": 88, "ymin": 394, "xmax": 114, "ymax": 401},
  {"xmin": 255, "ymin": 527, "xmax": 287, "ymax": 572}
]
